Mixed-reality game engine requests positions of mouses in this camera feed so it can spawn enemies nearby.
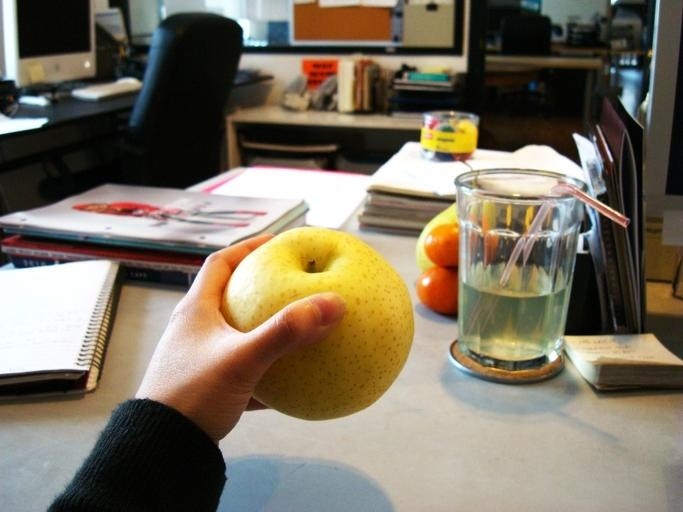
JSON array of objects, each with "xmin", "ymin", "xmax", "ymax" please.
[{"xmin": 116, "ymin": 77, "xmax": 140, "ymax": 83}]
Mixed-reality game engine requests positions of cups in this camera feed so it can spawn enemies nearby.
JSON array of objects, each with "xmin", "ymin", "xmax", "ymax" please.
[
  {"xmin": 420, "ymin": 110, "xmax": 480, "ymax": 161},
  {"xmin": 455, "ymin": 167, "xmax": 589, "ymax": 370}
]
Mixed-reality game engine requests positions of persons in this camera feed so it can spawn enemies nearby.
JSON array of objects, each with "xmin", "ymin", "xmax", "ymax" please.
[
  {"xmin": 73, "ymin": 200, "xmax": 269, "ymax": 228},
  {"xmin": 45, "ymin": 231, "xmax": 347, "ymax": 509}
]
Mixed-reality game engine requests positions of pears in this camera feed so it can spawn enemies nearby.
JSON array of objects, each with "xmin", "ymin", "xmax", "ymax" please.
[{"xmin": 221, "ymin": 226, "xmax": 414, "ymax": 421}]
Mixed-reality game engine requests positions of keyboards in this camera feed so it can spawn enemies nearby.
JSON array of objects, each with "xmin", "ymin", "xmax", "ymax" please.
[{"xmin": 71, "ymin": 83, "xmax": 142, "ymax": 104}]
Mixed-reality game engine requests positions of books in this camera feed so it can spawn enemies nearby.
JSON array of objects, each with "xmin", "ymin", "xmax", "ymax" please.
[
  {"xmin": 335, "ymin": 57, "xmax": 380, "ymax": 112},
  {"xmin": 0, "ymin": 183, "xmax": 308, "ymax": 289},
  {"xmin": 0, "ymin": 257, "xmax": 122, "ymax": 405},
  {"xmin": 357, "ymin": 138, "xmax": 552, "ymax": 233}
]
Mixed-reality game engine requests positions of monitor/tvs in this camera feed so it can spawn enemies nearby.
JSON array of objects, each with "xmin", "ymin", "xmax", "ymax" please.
[{"xmin": 0, "ymin": 0, "xmax": 97, "ymax": 106}]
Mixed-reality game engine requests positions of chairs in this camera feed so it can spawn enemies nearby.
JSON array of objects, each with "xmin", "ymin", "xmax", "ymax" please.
[{"xmin": 35, "ymin": 11, "xmax": 243, "ymax": 202}]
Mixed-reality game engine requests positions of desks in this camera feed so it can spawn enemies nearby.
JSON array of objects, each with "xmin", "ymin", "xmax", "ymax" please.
[
  {"xmin": 0, "ymin": 167, "xmax": 681, "ymax": 512},
  {"xmin": 226, "ymin": 104, "xmax": 428, "ymax": 169},
  {"xmin": 0, "ymin": 69, "xmax": 274, "ymax": 264},
  {"xmin": 484, "ymin": 56, "xmax": 604, "ymax": 165},
  {"xmin": 550, "ymin": 42, "xmax": 645, "ymax": 56}
]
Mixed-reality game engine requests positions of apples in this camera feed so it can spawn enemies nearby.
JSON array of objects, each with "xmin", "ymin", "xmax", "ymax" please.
[
  {"xmin": 420, "ymin": 219, "xmax": 459, "ymax": 268},
  {"xmin": 417, "ymin": 269, "xmax": 458, "ymax": 316}
]
